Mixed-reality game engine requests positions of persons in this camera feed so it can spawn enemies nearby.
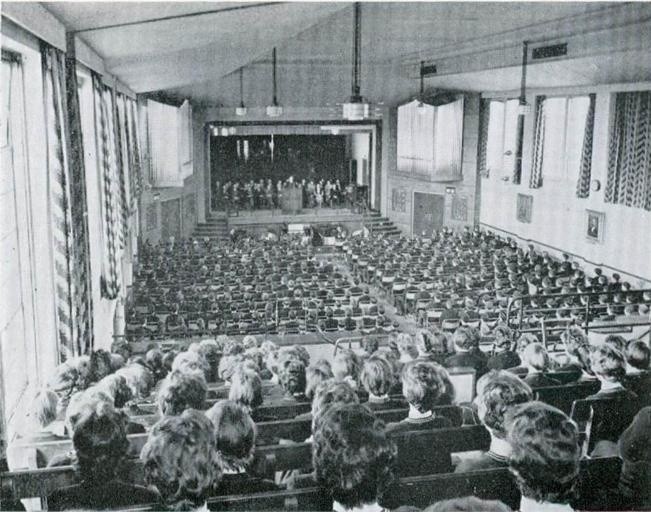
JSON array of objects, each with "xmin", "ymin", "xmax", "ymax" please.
[
  {"xmin": 127, "ymin": 222, "xmax": 402, "ymax": 340},
  {"xmin": 212, "ymin": 176, "xmax": 349, "ymax": 211},
  {"xmin": 341, "ymin": 221, "xmax": 651, "ymax": 330},
  {"xmin": 2, "ymin": 326, "xmax": 651, "ymax": 511}
]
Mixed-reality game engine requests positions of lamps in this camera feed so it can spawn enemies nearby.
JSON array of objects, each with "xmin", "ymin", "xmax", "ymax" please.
[
  {"xmin": 235, "ymin": 67, "xmax": 247, "ymax": 116},
  {"xmin": 266, "ymin": 47, "xmax": 282, "ymax": 117},
  {"xmin": 342, "ymin": 1, "xmax": 369, "ymax": 121}
]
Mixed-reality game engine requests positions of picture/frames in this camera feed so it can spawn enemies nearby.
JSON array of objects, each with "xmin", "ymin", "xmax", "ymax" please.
[{"xmin": 585, "ymin": 209, "xmax": 605, "ymax": 243}]
